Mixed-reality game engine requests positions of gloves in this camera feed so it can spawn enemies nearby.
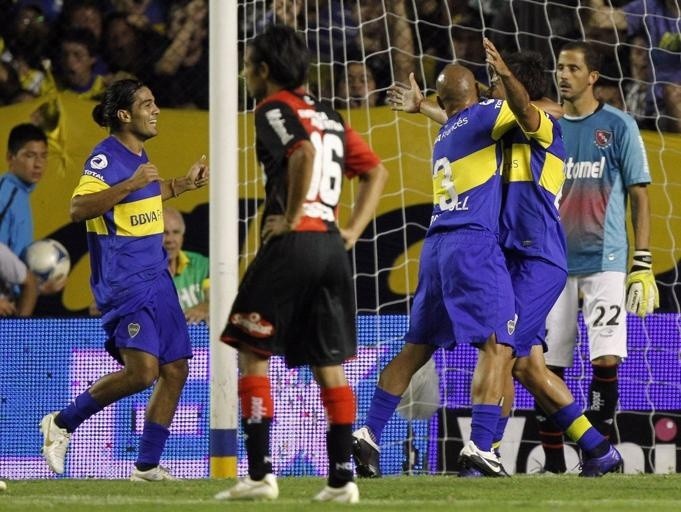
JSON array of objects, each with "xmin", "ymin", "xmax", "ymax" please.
[{"xmin": 625, "ymin": 250, "xmax": 660, "ymax": 319}]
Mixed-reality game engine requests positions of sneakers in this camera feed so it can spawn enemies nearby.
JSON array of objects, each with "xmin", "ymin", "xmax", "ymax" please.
[
  {"xmin": 39, "ymin": 411, "xmax": 72, "ymax": 474},
  {"xmin": 129, "ymin": 464, "xmax": 178, "ymax": 483},
  {"xmin": 458, "ymin": 440, "xmax": 623, "ymax": 477},
  {"xmin": 353, "ymin": 426, "xmax": 381, "ymax": 476},
  {"xmin": 312, "ymin": 480, "xmax": 359, "ymax": 505},
  {"xmin": 214, "ymin": 473, "xmax": 278, "ymax": 501}
]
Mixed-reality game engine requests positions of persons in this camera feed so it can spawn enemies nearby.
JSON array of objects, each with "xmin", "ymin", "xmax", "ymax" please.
[
  {"xmin": 0, "ymin": 122, "xmax": 67, "ymax": 299},
  {"xmin": 239, "ymin": 1, "xmax": 680, "ymax": 133},
  {"xmin": 349, "ymin": 63, "xmax": 563, "ymax": 479},
  {"xmin": 0, "ymin": 0, "xmax": 209, "ymax": 113},
  {"xmin": 0, "ymin": 280, "xmax": 1, "ymax": 316},
  {"xmin": 215, "ymin": 21, "xmax": 389, "ymax": 504},
  {"xmin": 531, "ymin": 41, "xmax": 661, "ymax": 479},
  {"xmin": 160, "ymin": 205, "xmax": 209, "ymax": 325},
  {"xmin": 38, "ymin": 78, "xmax": 209, "ymax": 483},
  {"xmin": 385, "ymin": 37, "xmax": 622, "ymax": 478}
]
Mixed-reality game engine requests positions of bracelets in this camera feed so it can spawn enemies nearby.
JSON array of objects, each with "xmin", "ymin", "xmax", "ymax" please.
[
  {"xmin": 172, "ymin": 177, "xmax": 179, "ymax": 198},
  {"xmin": 630, "ymin": 248, "xmax": 652, "ymax": 269}
]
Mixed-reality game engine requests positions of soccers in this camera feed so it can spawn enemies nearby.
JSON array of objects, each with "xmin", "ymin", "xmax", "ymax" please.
[{"xmin": 19, "ymin": 237, "xmax": 72, "ymax": 283}]
